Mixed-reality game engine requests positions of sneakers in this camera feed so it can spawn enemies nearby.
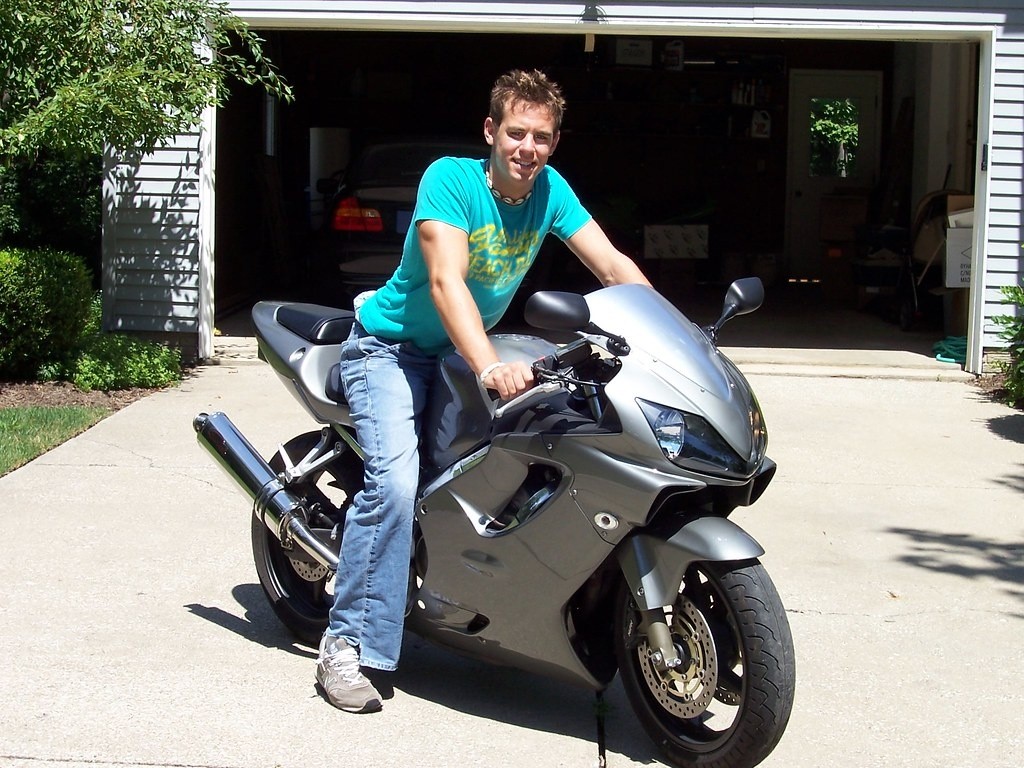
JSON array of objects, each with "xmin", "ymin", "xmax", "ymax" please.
[{"xmin": 316, "ymin": 634, "xmax": 383, "ymax": 714}]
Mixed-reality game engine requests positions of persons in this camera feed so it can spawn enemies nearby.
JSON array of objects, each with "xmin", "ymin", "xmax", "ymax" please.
[{"xmin": 315, "ymin": 70, "xmax": 656, "ymax": 715}]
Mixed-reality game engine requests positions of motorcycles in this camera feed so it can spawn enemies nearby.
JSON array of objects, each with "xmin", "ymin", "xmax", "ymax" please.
[{"xmin": 190, "ymin": 273, "xmax": 798, "ymax": 768}]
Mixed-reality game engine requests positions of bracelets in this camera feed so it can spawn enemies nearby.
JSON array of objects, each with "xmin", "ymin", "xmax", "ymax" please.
[{"xmin": 480, "ymin": 362, "xmax": 506, "ymax": 391}]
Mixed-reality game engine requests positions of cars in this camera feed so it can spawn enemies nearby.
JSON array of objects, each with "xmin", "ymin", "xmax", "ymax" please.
[{"xmin": 313, "ymin": 141, "xmax": 495, "ymax": 312}]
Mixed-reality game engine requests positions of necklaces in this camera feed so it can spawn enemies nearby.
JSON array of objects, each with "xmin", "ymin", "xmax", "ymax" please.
[{"xmin": 486, "ymin": 159, "xmax": 532, "ymax": 206}]
